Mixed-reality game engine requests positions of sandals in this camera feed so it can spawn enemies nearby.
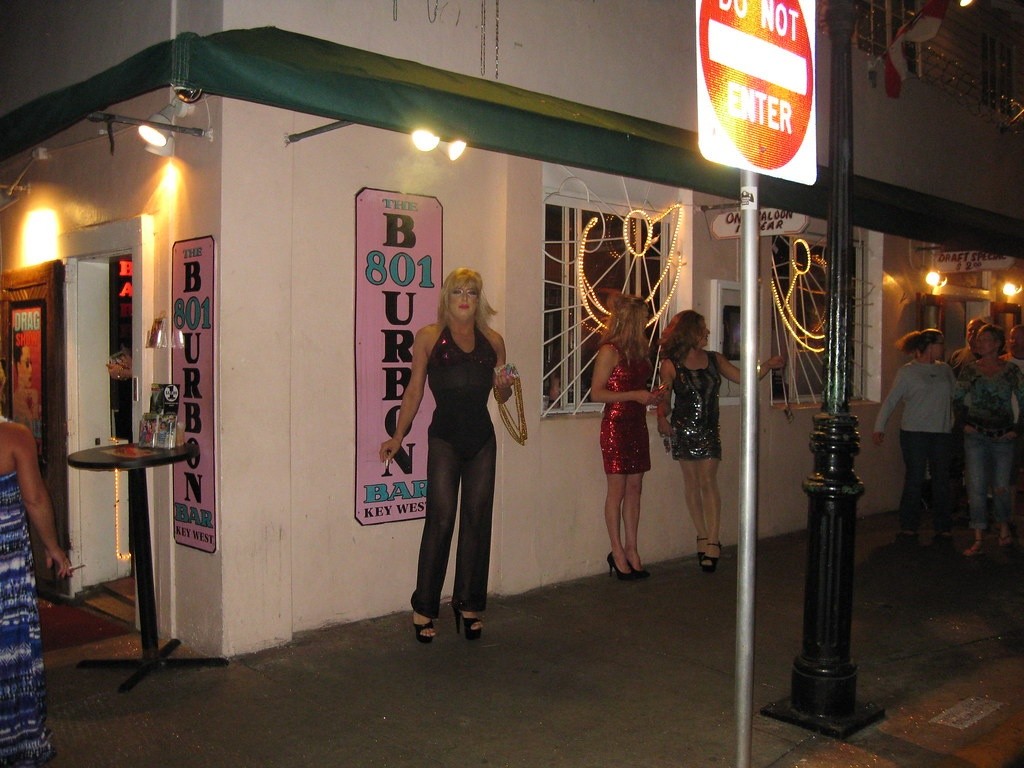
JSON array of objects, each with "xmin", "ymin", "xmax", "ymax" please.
[
  {"xmin": 962, "ymin": 539, "xmax": 986, "ymax": 560},
  {"xmin": 998, "ymin": 524, "xmax": 1014, "ymax": 546}
]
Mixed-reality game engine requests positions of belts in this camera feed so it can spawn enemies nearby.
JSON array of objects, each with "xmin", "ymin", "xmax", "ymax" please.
[{"xmin": 969, "ymin": 421, "xmax": 1013, "ymax": 438}]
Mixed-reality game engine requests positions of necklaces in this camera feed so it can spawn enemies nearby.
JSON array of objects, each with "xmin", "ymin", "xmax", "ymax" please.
[{"xmin": 494, "ymin": 374, "xmax": 528, "ymax": 446}]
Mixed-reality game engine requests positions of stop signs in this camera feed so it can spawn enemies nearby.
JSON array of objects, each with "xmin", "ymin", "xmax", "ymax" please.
[{"xmin": 700, "ymin": 0, "xmax": 817, "ymax": 171}]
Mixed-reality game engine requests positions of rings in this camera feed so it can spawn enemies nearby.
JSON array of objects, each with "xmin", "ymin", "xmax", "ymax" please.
[{"xmin": 661, "ymin": 434, "xmax": 665, "ymax": 437}]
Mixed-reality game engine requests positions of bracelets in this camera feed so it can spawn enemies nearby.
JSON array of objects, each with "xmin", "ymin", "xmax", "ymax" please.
[{"xmin": 117, "ymin": 365, "xmax": 130, "ymax": 380}]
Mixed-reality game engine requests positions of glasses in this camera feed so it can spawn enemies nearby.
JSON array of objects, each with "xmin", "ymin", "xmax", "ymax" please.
[{"xmin": 929, "ymin": 341, "xmax": 945, "ymax": 349}]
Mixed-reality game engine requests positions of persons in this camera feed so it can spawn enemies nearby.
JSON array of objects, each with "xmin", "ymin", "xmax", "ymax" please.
[
  {"xmin": 140, "ymin": 421, "xmax": 148, "ymax": 447},
  {"xmin": 656, "ymin": 311, "xmax": 786, "ymax": 573},
  {"xmin": 379, "ymin": 267, "xmax": 512, "ymax": 644},
  {"xmin": 146, "ymin": 426, "xmax": 152, "ymax": 445},
  {"xmin": 955, "ymin": 323, "xmax": 1024, "ymax": 560},
  {"xmin": 547, "ymin": 341, "xmax": 589, "ymax": 408},
  {"xmin": 872, "ymin": 330, "xmax": 956, "ymax": 553},
  {"xmin": 165, "ymin": 416, "xmax": 175, "ymax": 447},
  {"xmin": 106, "ymin": 354, "xmax": 133, "ymax": 380},
  {"xmin": 591, "ymin": 287, "xmax": 663, "ymax": 581},
  {"xmin": 158, "ymin": 422, "xmax": 167, "ymax": 448},
  {"xmin": 12, "ymin": 326, "xmax": 41, "ymax": 435},
  {"xmin": 0, "ymin": 366, "xmax": 72, "ymax": 768},
  {"xmin": 948, "ymin": 319, "xmax": 1024, "ymax": 535}
]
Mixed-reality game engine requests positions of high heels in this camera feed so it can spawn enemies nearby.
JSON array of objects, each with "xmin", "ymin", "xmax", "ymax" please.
[
  {"xmin": 701, "ymin": 541, "xmax": 723, "ymax": 572},
  {"xmin": 696, "ymin": 535, "xmax": 709, "ymax": 565},
  {"xmin": 607, "ymin": 552, "xmax": 636, "ymax": 581},
  {"xmin": 627, "ymin": 559, "xmax": 652, "ymax": 579},
  {"xmin": 452, "ymin": 606, "xmax": 482, "ymax": 640},
  {"xmin": 412, "ymin": 610, "xmax": 435, "ymax": 645}
]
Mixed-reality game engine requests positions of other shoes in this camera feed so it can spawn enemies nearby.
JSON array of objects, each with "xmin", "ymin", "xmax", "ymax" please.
[
  {"xmin": 934, "ymin": 531, "xmax": 952, "ymax": 543},
  {"xmin": 897, "ymin": 532, "xmax": 920, "ymax": 544}
]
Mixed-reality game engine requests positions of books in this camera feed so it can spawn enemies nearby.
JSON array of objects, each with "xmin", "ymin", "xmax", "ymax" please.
[{"xmin": 138, "ymin": 413, "xmax": 177, "ymax": 449}]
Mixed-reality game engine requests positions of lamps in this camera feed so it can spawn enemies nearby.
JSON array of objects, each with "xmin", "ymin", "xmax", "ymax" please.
[
  {"xmin": 138, "ymin": 98, "xmax": 187, "ymax": 159},
  {"xmin": 412, "ymin": 129, "xmax": 466, "ymax": 161},
  {"xmin": 992, "ymin": 273, "xmax": 1022, "ymax": 296},
  {"xmin": 918, "ymin": 264, "xmax": 948, "ymax": 289}
]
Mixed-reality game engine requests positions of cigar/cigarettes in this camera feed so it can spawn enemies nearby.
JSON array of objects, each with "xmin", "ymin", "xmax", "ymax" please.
[
  {"xmin": 386, "ymin": 460, "xmax": 388, "ymax": 467},
  {"xmin": 69, "ymin": 564, "xmax": 85, "ymax": 570}
]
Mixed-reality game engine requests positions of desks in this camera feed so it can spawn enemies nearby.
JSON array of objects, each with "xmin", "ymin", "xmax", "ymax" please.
[{"xmin": 67, "ymin": 444, "xmax": 230, "ymax": 694}]
